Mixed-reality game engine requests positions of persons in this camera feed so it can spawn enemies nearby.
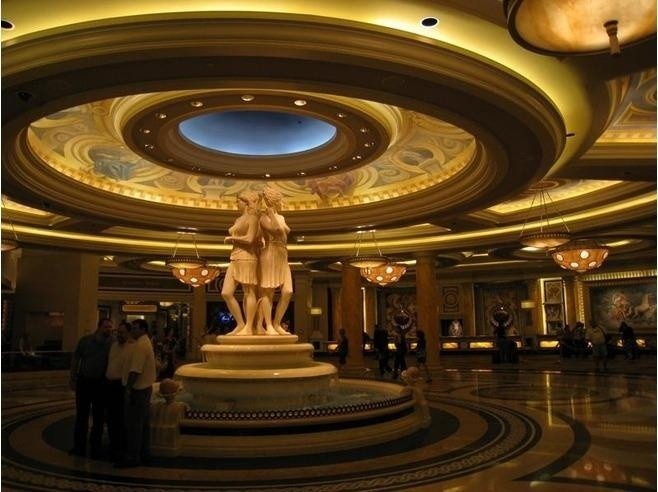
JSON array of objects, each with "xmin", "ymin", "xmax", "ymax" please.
[
  {"xmin": 102, "ymin": 319, "xmax": 132, "ymax": 457},
  {"xmin": 522, "ymin": 322, "xmax": 638, "ymax": 376},
  {"xmin": 220, "ymin": 187, "xmax": 294, "ymax": 338},
  {"xmin": 493, "ymin": 322, "xmax": 512, "ymax": 337},
  {"xmin": 497, "ymin": 337, "xmax": 520, "ymax": 379},
  {"xmin": 158, "ymin": 378, "xmax": 186, "ymax": 416},
  {"xmin": 110, "ymin": 318, "xmax": 219, "ymax": 377},
  {"xmin": 69, "ymin": 315, "xmax": 113, "ymax": 458},
  {"xmin": 332, "ymin": 329, "xmax": 352, "ymax": 370},
  {"xmin": 363, "ymin": 322, "xmax": 433, "ymax": 383},
  {"xmin": 18, "ymin": 333, "xmax": 33, "ymax": 357},
  {"xmin": 113, "ymin": 317, "xmax": 155, "ymax": 469}
]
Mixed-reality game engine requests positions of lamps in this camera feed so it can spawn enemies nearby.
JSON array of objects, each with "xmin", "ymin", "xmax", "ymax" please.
[
  {"xmin": 360, "ymin": 260, "xmax": 408, "ymax": 287},
  {"xmin": 519, "ymin": 180, "xmax": 572, "ymax": 256},
  {"xmin": 164, "ymin": 230, "xmax": 206, "ymax": 276},
  {"xmin": 502, "ymin": 1, "xmax": 657, "ymax": 60},
  {"xmin": 346, "ymin": 224, "xmax": 388, "ymax": 268},
  {"xmin": 172, "ymin": 262, "xmax": 222, "ymax": 288},
  {"xmin": 552, "ymin": 236, "xmax": 611, "ymax": 273}
]
